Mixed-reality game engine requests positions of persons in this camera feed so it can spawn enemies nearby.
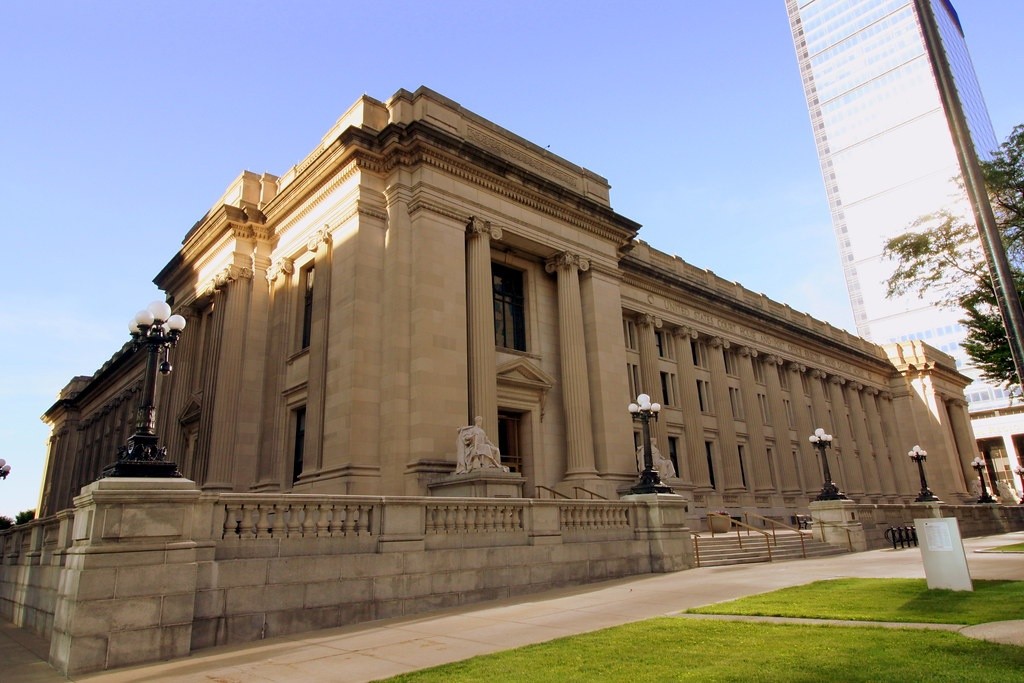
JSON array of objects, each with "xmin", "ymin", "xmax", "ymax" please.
[
  {"xmin": 650, "ymin": 438, "xmax": 676, "ymax": 480},
  {"xmin": 461, "ymin": 416, "xmax": 510, "ymax": 473}
]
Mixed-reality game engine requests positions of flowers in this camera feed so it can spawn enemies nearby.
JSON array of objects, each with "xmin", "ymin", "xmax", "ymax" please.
[{"xmin": 707, "ymin": 510, "xmax": 730, "ymax": 515}]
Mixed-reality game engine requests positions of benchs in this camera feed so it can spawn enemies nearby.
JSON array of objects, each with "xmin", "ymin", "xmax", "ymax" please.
[{"xmin": 794, "ymin": 512, "xmax": 813, "ymax": 530}]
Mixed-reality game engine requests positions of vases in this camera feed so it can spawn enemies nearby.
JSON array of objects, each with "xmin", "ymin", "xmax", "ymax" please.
[{"xmin": 707, "ymin": 514, "xmax": 731, "ymax": 533}]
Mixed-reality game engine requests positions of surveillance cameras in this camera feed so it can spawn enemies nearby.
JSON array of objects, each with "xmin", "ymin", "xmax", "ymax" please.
[{"xmin": 159, "ymin": 362, "xmax": 172, "ymax": 376}]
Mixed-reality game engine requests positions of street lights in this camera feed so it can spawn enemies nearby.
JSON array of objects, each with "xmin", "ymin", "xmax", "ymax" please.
[
  {"xmin": 1014, "ymin": 463, "xmax": 1024, "ymax": 506},
  {"xmin": 971, "ymin": 457, "xmax": 999, "ymax": 503},
  {"xmin": 616, "ymin": 393, "xmax": 675, "ymax": 494},
  {"xmin": 807, "ymin": 427, "xmax": 848, "ymax": 500},
  {"xmin": 89, "ymin": 300, "xmax": 189, "ymax": 479},
  {"xmin": 907, "ymin": 443, "xmax": 942, "ymax": 501}
]
